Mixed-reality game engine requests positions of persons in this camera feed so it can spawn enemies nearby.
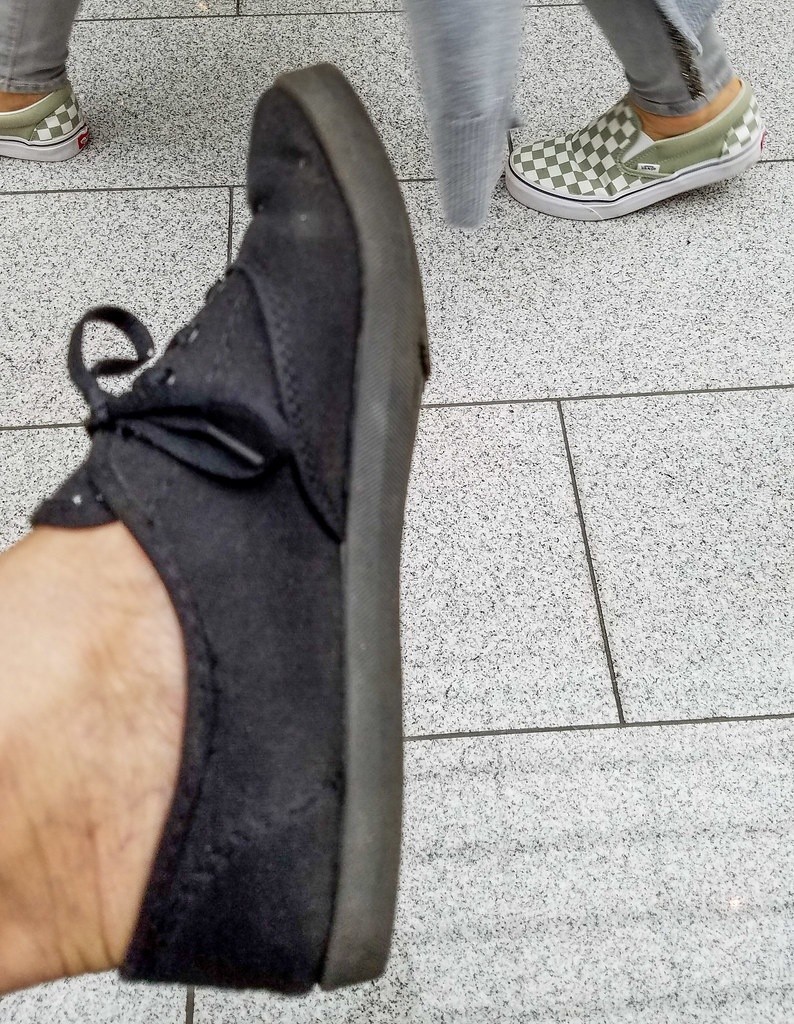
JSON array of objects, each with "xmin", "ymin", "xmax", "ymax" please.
[
  {"xmin": 2, "ymin": 64, "xmax": 434, "ymax": 999},
  {"xmin": 0, "ymin": 0, "xmax": 761, "ymax": 233}
]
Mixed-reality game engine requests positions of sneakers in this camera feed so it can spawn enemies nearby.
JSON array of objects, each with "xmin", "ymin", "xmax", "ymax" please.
[{"xmin": 31, "ymin": 70, "xmax": 430, "ymax": 992}]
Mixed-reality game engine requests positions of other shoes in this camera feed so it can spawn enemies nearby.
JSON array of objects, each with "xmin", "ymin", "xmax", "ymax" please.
[
  {"xmin": 0, "ymin": 73, "xmax": 89, "ymax": 164},
  {"xmin": 508, "ymin": 75, "xmax": 764, "ymax": 220}
]
